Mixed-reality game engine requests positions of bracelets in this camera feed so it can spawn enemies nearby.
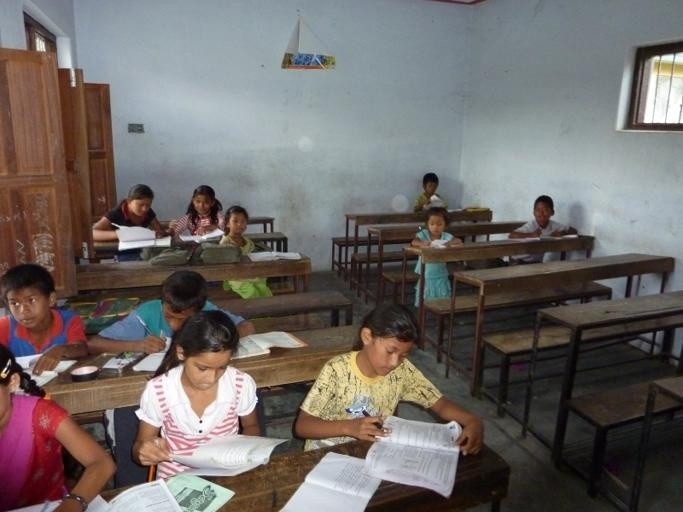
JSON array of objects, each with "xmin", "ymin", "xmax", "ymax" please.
[{"xmin": 61, "ymin": 490, "xmax": 88, "ymax": 508}]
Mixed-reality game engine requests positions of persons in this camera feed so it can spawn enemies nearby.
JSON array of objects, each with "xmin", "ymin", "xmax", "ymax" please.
[
  {"xmin": 416, "ymin": 172, "xmax": 444, "ymax": 210},
  {"xmin": 129, "ymin": 310, "xmax": 264, "ymax": 484},
  {"xmin": 86, "ymin": 268, "xmax": 255, "ymax": 491},
  {"xmin": 412, "ymin": 207, "xmax": 464, "ymax": 310},
  {"xmin": 90, "ymin": 183, "xmax": 163, "ymax": 241},
  {"xmin": 0, "ymin": 345, "xmax": 119, "ymax": 512},
  {"xmin": 0, "ymin": 264, "xmax": 87, "ymax": 374},
  {"xmin": 294, "ymin": 298, "xmax": 484, "ymax": 458},
  {"xmin": 500, "ymin": 194, "xmax": 579, "ymax": 268},
  {"xmin": 219, "ymin": 204, "xmax": 274, "ymax": 300},
  {"xmin": 167, "ymin": 184, "xmax": 225, "ymax": 246}
]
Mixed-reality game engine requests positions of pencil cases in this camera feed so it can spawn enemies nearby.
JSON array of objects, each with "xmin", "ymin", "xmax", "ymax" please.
[{"xmin": 102, "ymin": 352, "xmax": 144, "ymax": 374}]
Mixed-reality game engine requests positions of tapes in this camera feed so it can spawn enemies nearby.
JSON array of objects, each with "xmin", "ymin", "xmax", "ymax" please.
[{"xmin": 70, "ymin": 365, "xmax": 98, "ymax": 381}]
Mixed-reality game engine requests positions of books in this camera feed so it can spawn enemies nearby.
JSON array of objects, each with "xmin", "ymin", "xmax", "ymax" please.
[
  {"xmin": 164, "ymin": 472, "xmax": 235, "ymax": 512},
  {"xmin": 277, "ymin": 448, "xmax": 381, "ymax": 511},
  {"xmin": 368, "ymin": 413, "xmax": 464, "ymax": 497},
  {"xmin": 178, "ymin": 226, "xmax": 229, "ymax": 242},
  {"xmin": 83, "ymin": 477, "xmax": 183, "ymax": 512},
  {"xmin": 14, "ymin": 353, "xmax": 76, "ymax": 372},
  {"xmin": 228, "ymin": 329, "xmax": 307, "ymax": 363},
  {"xmin": 111, "ymin": 223, "xmax": 171, "ymax": 251},
  {"xmin": 248, "ymin": 249, "xmax": 302, "ymax": 262},
  {"xmin": 131, "ymin": 336, "xmax": 169, "ymax": 372},
  {"xmin": 167, "ymin": 436, "xmax": 288, "ymax": 477},
  {"xmin": 18, "ymin": 369, "xmax": 57, "ymax": 386}
]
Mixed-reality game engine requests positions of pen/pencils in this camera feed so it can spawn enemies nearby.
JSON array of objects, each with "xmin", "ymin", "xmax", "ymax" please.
[
  {"xmin": 111, "ymin": 222, "xmax": 120, "ymax": 228},
  {"xmin": 41, "ymin": 502, "xmax": 49, "ymax": 512},
  {"xmin": 148, "ymin": 440, "xmax": 159, "ymax": 482},
  {"xmin": 135, "ymin": 316, "xmax": 152, "ymax": 334},
  {"xmin": 418, "ymin": 225, "xmax": 429, "ymax": 241},
  {"xmin": 362, "ymin": 410, "xmax": 387, "ymax": 433}
]
